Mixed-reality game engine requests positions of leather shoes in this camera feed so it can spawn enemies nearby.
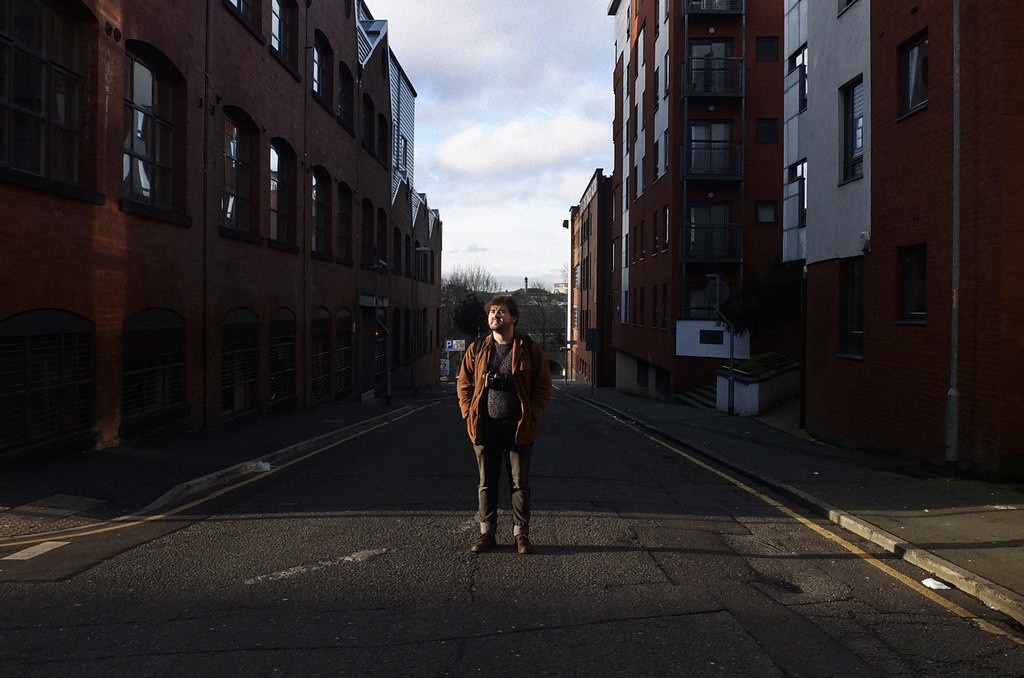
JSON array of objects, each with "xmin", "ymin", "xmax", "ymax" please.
[
  {"xmin": 470, "ymin": 534, "xmax": 496, "ymax": 552},
  {"xmin": 514, "ymin": 533, "xmax": 531, "ymax": 554}
]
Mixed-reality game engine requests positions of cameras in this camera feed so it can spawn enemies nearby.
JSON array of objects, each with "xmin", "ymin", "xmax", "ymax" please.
[{"xmin": 484, "ymin": 371, "xmax": 510, "ymax": 392}]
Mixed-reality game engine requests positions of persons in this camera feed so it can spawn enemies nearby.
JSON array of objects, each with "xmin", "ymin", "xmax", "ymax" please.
[{"xmin": 455, "ymin": 297, "xmax": 552, "ymax": 555}]
